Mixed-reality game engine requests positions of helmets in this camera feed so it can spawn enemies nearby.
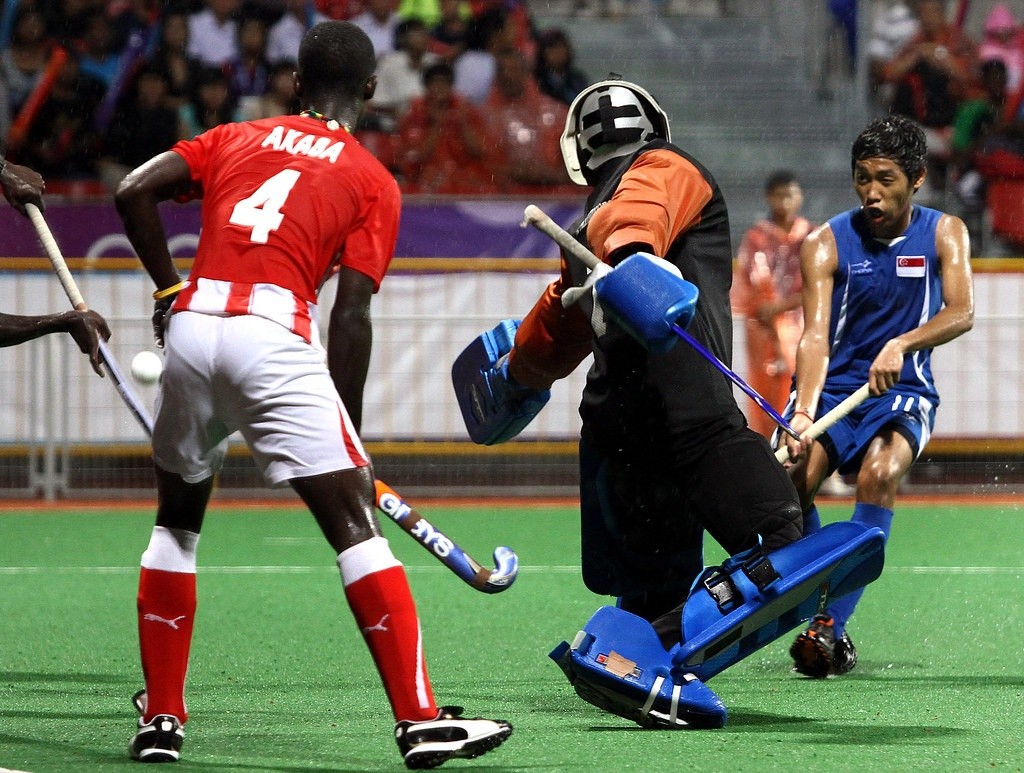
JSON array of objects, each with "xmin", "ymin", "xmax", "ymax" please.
[{"xmin": 559, "ymin": 79, "xmax": 672, "ymax": 184}]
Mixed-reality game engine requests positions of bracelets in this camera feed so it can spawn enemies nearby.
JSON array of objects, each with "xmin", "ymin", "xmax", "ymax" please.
[
  {"xmin": 791, "ymin": 410, "xmax": 816, "ymax": 423},
  {"xmin": 153, "ymin": 278, "xmax": 186, "ymax": 301}
]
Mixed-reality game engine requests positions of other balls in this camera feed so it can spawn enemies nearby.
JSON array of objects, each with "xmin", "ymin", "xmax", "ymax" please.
[{"xmin": 132, "ymin": 350, "xmax": 163, "ymax": 384}]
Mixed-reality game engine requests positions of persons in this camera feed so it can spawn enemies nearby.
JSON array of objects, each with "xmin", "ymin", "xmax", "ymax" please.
[
  {"xmin": 727, "ymin": 166, "xmax": 834, "ymax": 441},
  {"xmin": 446, "ymin": 79, "xmax": 858, "ymax": 677},
  {"xmin": 771, "ymin": 110, "xmax": 977, "ymax": 685},
  {"xmin": 870, "ymin": 0, "xmax": 1024, "ymax": 256},
  {"xmin": 0, "ymin": 0, "xmax": 599, "ymax": 185},
  {"xmin": 112, "ymin": 19, "xmax": 514, "ymax": 772},
  {"xmin": 0, "ymin": 155, "xmax": 114, "ymax": 377}
]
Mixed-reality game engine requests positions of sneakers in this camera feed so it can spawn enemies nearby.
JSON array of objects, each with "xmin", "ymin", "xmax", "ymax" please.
[
  {"xmin": 127, "ymin": 689, "xmax": 185, "ymax": 761},
  {"xmin": 393, "ymin": 706, "xmax": 513, "ymax": 769}
]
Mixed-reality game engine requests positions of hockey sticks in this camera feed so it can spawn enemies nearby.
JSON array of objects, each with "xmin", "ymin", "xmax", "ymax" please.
[
  {"xmin": 25, "ymin": 202, "xmax": 228, "ymax": 476},
  {"xmin": 151, "ymin": 308, "xmax": 518, "ymax": 594},
  {"xmin": 773, "ymin": 381, "xmax": 870, "ymax": 466},
  {"xmin": 524, "ymin": 203, "xmax": 804, "ymax": 441}
]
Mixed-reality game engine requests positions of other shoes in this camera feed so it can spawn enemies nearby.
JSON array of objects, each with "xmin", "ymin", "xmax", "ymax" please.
[{"xmin": 790, "ymin": 614, "xmax": 857, "ymax": 676}]
